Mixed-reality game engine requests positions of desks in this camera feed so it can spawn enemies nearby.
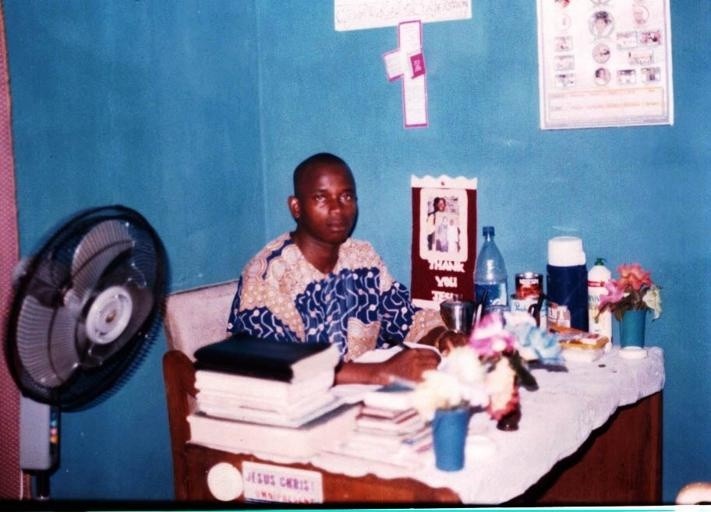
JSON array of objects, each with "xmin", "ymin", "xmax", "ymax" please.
[{"xmin": 192, "ymin": 345, "xmax": 664, "ymax": 506}]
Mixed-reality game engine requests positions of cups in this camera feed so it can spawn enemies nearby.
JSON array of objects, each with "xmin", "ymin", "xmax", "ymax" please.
[
  {"xmin": 440, "ymin": 295, "xmax": 474, "ymax": 335},
  {"xmin": 619, "ymin": 310, "xmax": 647, "ymax": 350}
]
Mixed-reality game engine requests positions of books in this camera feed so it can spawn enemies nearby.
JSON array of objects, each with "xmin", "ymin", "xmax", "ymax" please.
[{"xmin": 183, "ymin": 331, "xmax": 434, "ymax": 462}]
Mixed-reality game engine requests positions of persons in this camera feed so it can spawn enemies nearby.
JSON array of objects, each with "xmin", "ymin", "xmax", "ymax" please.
[
  {"xmin": 222, "ymin": 150, "xmax": 470, "ymax": 390},
  {"xmin": 553, "ymin": 0, "xmax": 660, "ymax": 87},
  {"xmin": 425, "ymin": 196, "xmax": 460, "ymax": 254}
]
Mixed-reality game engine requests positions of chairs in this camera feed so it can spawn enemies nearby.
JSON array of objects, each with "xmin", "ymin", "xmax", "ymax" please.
[{"xmin": 160, "ymin": 280, "xmax": 240, "ymax": 501}]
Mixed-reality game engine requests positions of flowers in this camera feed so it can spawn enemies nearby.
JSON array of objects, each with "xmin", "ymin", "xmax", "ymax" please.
[
  {"xmin": 591, "ymin": 262, "xmax": 665, "ymax": 327},
  {"xmin": 408, "ymin": 308, "xmax": 542, "ymax": 434}
]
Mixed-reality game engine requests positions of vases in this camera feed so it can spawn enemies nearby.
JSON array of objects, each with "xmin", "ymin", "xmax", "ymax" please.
[{"xmin": 618, "ymin": 310, "xmax": 648, "ymax": 359}]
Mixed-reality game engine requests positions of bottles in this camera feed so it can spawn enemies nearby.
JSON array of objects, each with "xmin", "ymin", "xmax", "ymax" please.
[
  {"xmin": 546, "ymin": 236, "xmax": 588, "ymax": 333},
  {"xmin": 473, "ymin": 225, "xmax": 510, "ymax": 313}
]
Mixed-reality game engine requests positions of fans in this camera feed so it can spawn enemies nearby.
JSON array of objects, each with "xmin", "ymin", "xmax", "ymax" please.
[{"xmin": 2, "ymin": 202, "xmax": 172, "ymax": 503}]
